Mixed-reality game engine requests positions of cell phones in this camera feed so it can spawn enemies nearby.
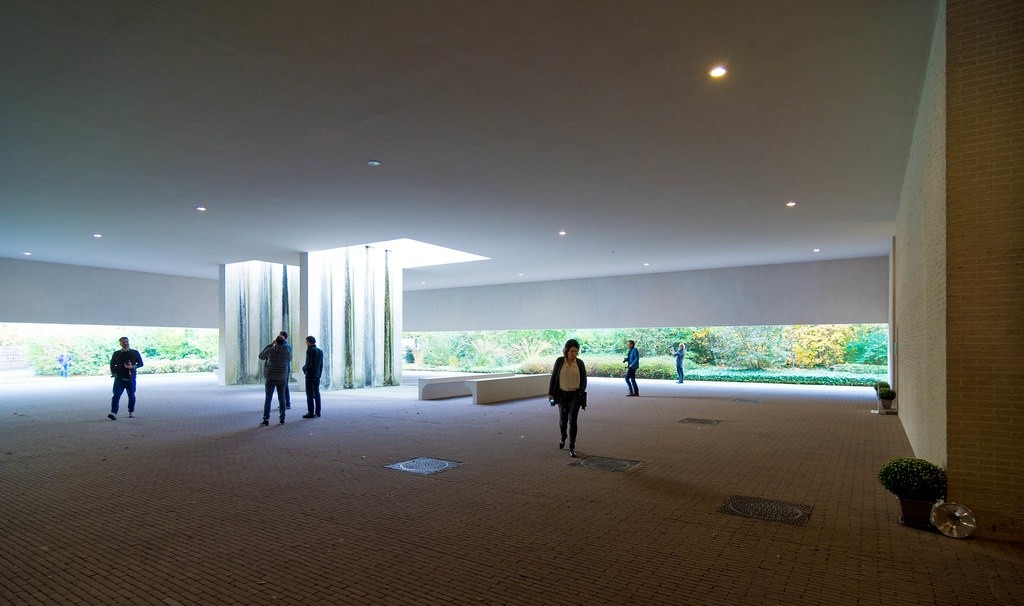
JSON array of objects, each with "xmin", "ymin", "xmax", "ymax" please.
[{"xmin": 550, "ymin": 399, "xmax": 555, "ymax": 406}]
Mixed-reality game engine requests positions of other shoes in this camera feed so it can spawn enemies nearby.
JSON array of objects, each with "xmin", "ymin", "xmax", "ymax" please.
[
  {"xmin": 260, "ymin": 420, "xmax": 268, "ymax": 426},
  {"xmin": 128, "ymin": 411, "xmax": 133, "ymax": 418},
  {"xmin": 286, "ymin": 404, "xmax": 290, "ymax": 409},
  {"xmin": 303, "ymin": 412, "xmax": 314, "ymax": 418},
  {"xmin": 570, "ymin": 447, "xmax": 576, "ymax": 458},
  {"xmin": 559, "ymin": 433, "xmax": 567, "ymax": 449},
  {"xmin": 677, "ymin": 381, "xmax": 683, "ymax": 384},
  {"xmin": 280, "ymin": 420, "xmax": 284, "ymax": 425},
  {"xmin": 627, "ymin": 392, "xmax": 639, "ymax": 397},
  {"xmin": 108, "ymin": 412, "xmax": 117, "ymax": 420},
  {"xmin": 315, "ymin": 411, "xmax": 320, "ymax": 417}
]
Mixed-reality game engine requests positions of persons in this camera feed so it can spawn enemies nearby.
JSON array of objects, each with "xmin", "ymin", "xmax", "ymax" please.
[
  {"xmin": 622, "ymin": 340, "xmax": 640, "ymax": 397},
  {"xmin": 107, "ymin": 336, "xmax": 144, "ymax": 421},
  {"xmin": 670, "ymin": 343, "xmax": 685, "ymax": 383},
  {"xmin": 302, "ymin": 336, "xmax": 323, "ymax": 418},
  {"xmin": 256, "ymin": 331, "xmax": 293, "ymax": 427},
  {"xmin": 548, "ymin": 339, "xmax": 587, "ymax": 457},
  {"xmin": 58, "ymin": 354, "xmax": 71, "ymax": 377}
]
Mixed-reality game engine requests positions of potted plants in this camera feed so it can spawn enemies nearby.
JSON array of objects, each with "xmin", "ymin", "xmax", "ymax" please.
[
  {"xmin": 874, "ymin": 382, "xmax": 890, "ymax": 392},
  {"xmin": 878, "ymin": 456, "xmax": 948, "ymax": 526},
  {"xmin": 877, "ymin": 388, "xmax": 896, "ymax": 410}
]
